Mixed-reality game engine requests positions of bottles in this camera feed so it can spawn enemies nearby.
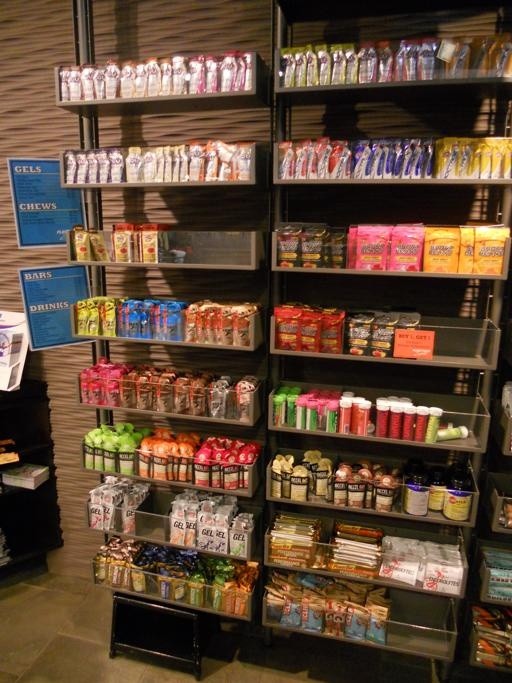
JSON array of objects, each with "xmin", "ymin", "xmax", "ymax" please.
[
  {"xmin": 401, "ymin": 458, "xmax": 472, "ymax": 521},
  {"xmin": 273, "ymin": 386, "xmax": 469, "ymax": 444}
]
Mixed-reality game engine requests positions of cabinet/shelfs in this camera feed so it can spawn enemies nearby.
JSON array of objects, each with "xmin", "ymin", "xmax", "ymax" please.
[
  {"xmin": 0, "ymin": 379, "xmax": 65, "ymax": 581},
  {"xmin": 56, "ymin": 0, "xmax": 512, "ymax": 683}
]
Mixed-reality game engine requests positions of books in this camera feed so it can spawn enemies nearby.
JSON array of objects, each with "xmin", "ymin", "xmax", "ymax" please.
[
  {"xmin": 0, "ymin": 527, "xmax": 12, "ymax": 567},
  {"xmin": 0, "ymin": 439, "xmax": 20, "ymax": 465},
  {"xmin": 2, "ymin": 462, "xmax": 50, "ymax": 490},
  {"xmin": 0, "ymin": 329, "xmax": 24, "ymax": 366}
]
